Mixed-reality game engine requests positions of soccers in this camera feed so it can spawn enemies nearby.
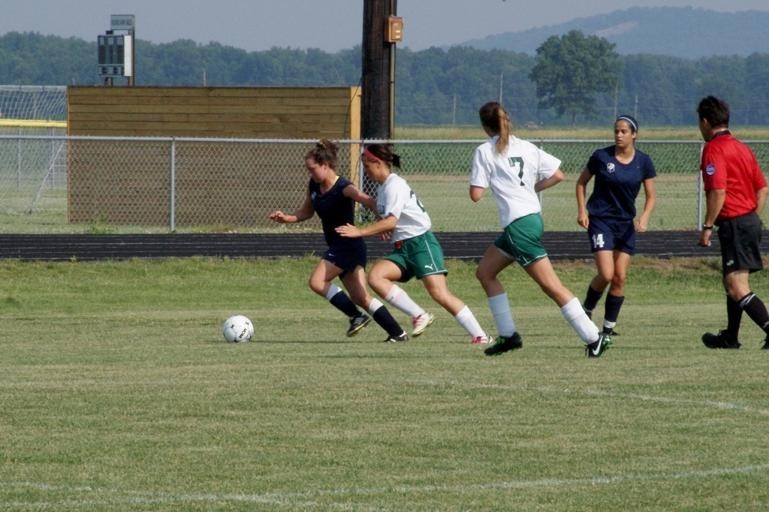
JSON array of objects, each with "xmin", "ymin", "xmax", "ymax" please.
[{"xmin": 223, "ymin": 314, "xmax": 254, "ymax": 343}]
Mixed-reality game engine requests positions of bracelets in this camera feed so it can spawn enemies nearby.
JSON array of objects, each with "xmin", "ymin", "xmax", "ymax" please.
[{"xmin": 701, "ymin": 224, "xmax": 714, "ymax": 229}]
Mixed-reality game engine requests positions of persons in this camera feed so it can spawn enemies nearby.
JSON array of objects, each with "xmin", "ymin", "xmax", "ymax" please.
[
  {"xmin": 696, "ymin": 96, "xmax": 769, "ymax": 349},
  {"xmin": 334, "ymin": 143, "xmax": 494, "ymax": 344},
  {"xmin": 269, "ymin": 138, "xmax": 409, "ymax": 343},
  {"xmin": 576, "ymin": 115, "xmax": 656, "ymax": 335},
  {"xmin": 469, "ymin": 102, "xmax": 613, "ymax": 358}
]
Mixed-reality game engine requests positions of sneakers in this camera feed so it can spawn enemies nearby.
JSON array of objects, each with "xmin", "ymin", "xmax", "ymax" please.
[
  {"xmin": 702, "ymin": 332, "xmax": 742, "ymax": 348},
  {"xmin": 346, "ymin": 306, "xmax": 620, "ymax": 357}
]
